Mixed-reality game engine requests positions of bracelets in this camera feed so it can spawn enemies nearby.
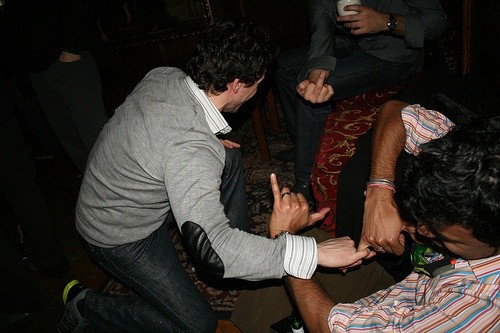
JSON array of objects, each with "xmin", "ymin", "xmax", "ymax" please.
[{"xmin": 366, "ymin": 178, "xmax": 397, "ymax": 193}]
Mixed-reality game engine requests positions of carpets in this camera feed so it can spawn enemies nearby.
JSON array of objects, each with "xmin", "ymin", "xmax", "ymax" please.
[{"xmin": 99, "ymin": 92, "xmax": 298, "ymax": 323}]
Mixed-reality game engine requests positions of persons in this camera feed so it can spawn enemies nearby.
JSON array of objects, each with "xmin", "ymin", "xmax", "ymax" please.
[
  {"xmin": 0, "ymin": 1, "xmax": 111, "ymax": 180},
  {"xmin": 48, "ymin": 17, "xmax": 369, "ymax": 333},
  {"xmin": 268, "ymin": 1, "xmax": 459, "ymax": 215},
  {"xmin": 231, "ymin": 98, "xmax": 500, "ymax": 333}
]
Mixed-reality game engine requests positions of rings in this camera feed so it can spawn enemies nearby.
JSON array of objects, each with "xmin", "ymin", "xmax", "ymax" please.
[
  {"xmin": 377, "ymin": 246, "xmax": 383, "ymax": 253},
  {"xmin": 281, "ymin": 193, "xmax": 291, "ymax": 198}
]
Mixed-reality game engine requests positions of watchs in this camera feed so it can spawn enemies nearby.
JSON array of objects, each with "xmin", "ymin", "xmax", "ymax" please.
[
  {"xmin": 387, "ymin": 12, "xmax": 398, "ymax": 33},
  {"xmin": 272, "ymin": 231, "xmax": 295, "ymax": 240}
]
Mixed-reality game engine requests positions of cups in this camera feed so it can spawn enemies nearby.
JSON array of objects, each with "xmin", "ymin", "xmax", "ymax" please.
[{"xmin": 337, "ymin": 0, "xmax": 361, "ymax": 19}]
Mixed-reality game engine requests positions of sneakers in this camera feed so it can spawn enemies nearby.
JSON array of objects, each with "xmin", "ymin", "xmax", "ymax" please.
[{"xmin": 51, "ymin": 274, "xmax": 94, "ymax": 333}]
